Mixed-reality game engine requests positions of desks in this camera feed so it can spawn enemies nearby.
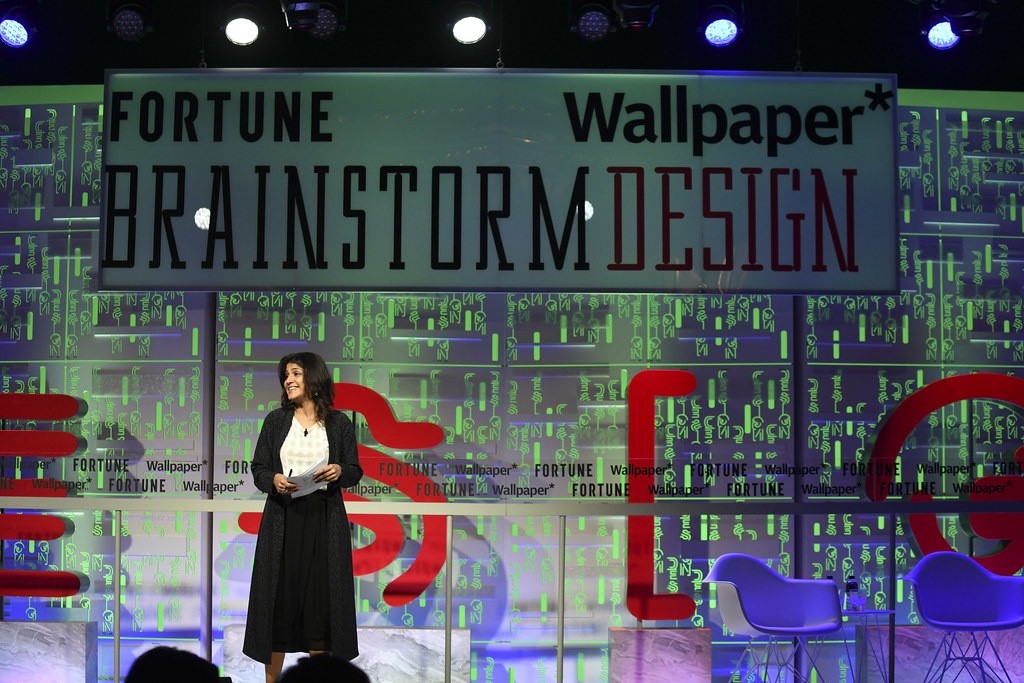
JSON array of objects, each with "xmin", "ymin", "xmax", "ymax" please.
[{"xmin": 839, "ymin": 610, "xmax": 897, "ymax": 683}]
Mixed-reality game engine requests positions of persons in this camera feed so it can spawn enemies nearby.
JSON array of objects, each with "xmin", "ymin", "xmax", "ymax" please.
[
  {"xmin": 278, "ymin": 652, "xmax": 374, "ymax": 683},
  {"xmin": 242, "ymin": 353, "xmax": 364, "ymax": 683},
  {"xmin": 124, "ymin": 646, "xmax": 232, "ymax": 683}
]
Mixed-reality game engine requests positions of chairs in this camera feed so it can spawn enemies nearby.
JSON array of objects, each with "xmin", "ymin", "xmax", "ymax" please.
[
  {"xmin": 702, "ymin": 553, "xmax": 842, "ymax": 683},
  {"xmin": 897, "ymin": 551, "xmax": 1024, "ymax": 683}
]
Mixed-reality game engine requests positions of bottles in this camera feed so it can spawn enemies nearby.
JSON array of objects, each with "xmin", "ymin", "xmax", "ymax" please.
[{"xmin": 846, "ymin": 575, "xmax": 860, "ymax": 611}]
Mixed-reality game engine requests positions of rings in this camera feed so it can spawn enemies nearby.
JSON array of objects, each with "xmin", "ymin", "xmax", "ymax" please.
[
  {"xmin": 282, "ymin": 491, "xmax": 285, "ymax": 495},
  {"xmin": 286, "ymin": 490, "xmax": 288, "ymax": 493}
]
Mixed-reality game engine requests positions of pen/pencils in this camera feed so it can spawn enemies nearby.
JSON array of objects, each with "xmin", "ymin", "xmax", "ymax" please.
[{"xmin": 288, "ymin": 469, "xmax": 293, "ymax": 478}]
